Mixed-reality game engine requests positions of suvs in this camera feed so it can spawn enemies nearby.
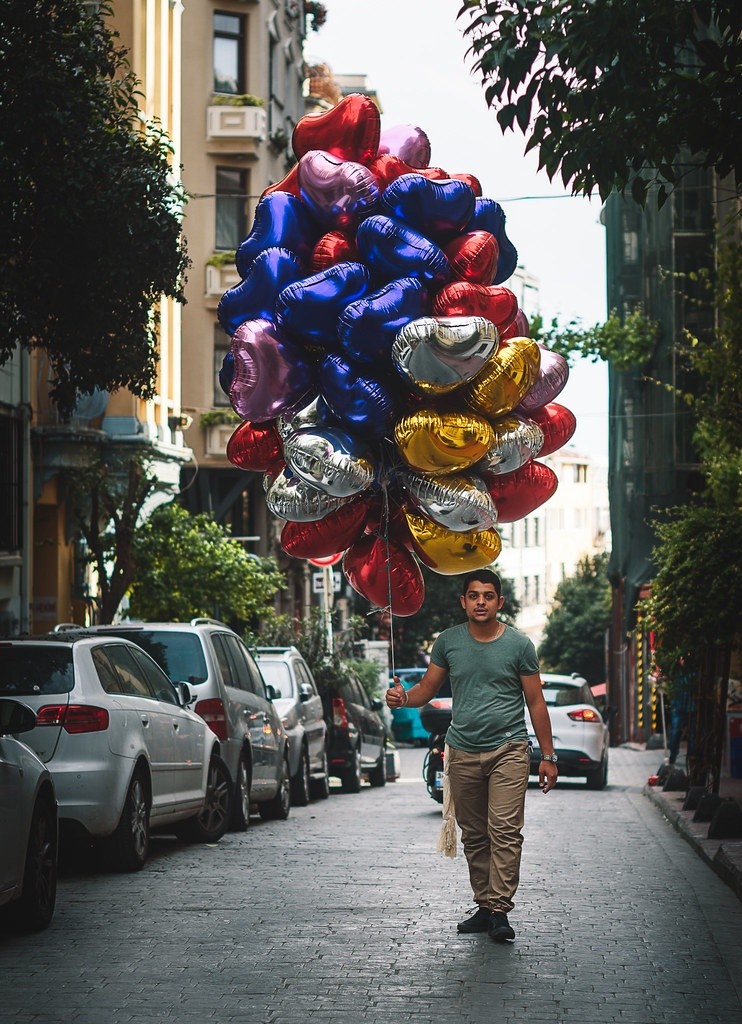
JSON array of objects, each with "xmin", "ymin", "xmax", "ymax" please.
[{"xmin": 523, "ymin": 672, "xmax": 611, "ymax": 791}]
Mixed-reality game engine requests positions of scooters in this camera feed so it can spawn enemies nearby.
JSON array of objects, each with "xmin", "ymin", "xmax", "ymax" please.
[{"xmin": 420, "ymin": 697, "xmax": 452, "ymax": 805}]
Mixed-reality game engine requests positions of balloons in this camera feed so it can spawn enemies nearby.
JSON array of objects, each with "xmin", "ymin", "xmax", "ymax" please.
[{"xmin": 218, "ymin": 93, "xmax": 577, "ymax": 617}]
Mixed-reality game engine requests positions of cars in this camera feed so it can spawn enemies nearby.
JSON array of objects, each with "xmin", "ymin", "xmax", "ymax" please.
[
  {"xmin": 388, "ymin": 667, "xmax": 452, "ymax": 750},
  {"xmin": 246, "ymin": 645, "xmax": 328, "ymax": 807},
  {"xmin": 306, "ymin": 658, "xmax": 388, "ymax": 793},
  {"xmin": 0, "ymin": 736, "xmax": 59, "ymax": 941},
  {"xmin": 0, "ymin": 632, "xmax": 234, "ymax": 874}
]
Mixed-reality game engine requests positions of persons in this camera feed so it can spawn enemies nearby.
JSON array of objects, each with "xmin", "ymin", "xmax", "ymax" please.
[{"xmin": 386, "ymin": 569, "xmax": 559, "ymax": 939}]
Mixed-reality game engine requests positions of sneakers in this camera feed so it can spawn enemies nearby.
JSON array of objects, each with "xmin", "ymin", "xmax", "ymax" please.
[
  {"xmin": 487, "ymin": 911, "xmax": 516, "ymax": 941},
  {"xmin": 457, "ymin": 908, "xmax": 492, "ymax": 933}
]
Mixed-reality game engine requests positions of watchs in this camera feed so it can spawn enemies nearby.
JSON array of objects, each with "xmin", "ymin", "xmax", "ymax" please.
[{"xmin": 541, "ymin": 752, "xmax": 558, "ymax": 762}]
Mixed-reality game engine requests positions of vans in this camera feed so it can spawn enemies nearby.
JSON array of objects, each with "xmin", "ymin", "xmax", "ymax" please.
[{"xmin": 53, "ymin": 616, "xmax": 292, "ymax": 832}]
